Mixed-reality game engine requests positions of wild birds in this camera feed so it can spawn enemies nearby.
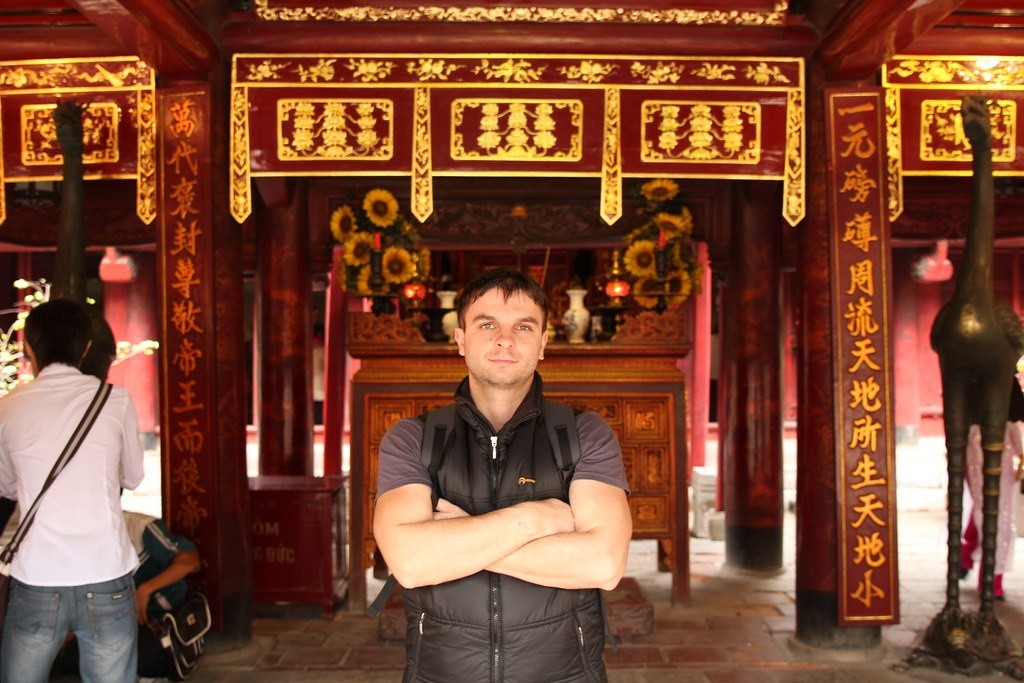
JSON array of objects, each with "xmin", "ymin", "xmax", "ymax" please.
[
  {"xmin": 52, "ymin": 96, "xmax": 93, "ymax": 318},
  {"xmin": 892, "ymin": 92, "xmax": 1024, "ymax": 683}
]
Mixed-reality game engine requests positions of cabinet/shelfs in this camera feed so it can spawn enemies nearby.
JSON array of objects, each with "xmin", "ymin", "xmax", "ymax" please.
[
  {"xmin": 247, "ymin": 476, "xmax": 353, "ymax": 618},
  {"xmin": 342, "ymin": 309, "xmax": 694, "ymax": 604}
]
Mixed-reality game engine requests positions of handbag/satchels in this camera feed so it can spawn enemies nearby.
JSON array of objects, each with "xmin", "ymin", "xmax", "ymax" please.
[
  {"xmin": 147, "ymin": 590, "xmax": 212, "ymax": 680},
  {"xmin": 0, "ymin": 502, "xmax": 15, "ymax": 627}
]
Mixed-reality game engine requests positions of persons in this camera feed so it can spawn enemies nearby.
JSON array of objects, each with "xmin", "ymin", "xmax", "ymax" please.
[
  {"xmin": 373, "ymin": 269, "xmax": 633, "ymax": 683},
  {"xmin": 0, "ymin": 299, "xmax": 145, "ymax": 683},
  {"xmin": 958, "ymin": 420, "xmax": 1024, "ymax": 601},
  {"xmin": 120, "ymin": 487, "xmax": 207, "ymax": 628}
]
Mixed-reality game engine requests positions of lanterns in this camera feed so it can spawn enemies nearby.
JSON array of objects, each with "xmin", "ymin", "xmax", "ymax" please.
[
  {"xmin": 404, "ymin": 280, "xmax": 426, "ymax": 306},
  {"xmin": 605, "ymin": 279, "xmax": 631, "ymax": 302}
]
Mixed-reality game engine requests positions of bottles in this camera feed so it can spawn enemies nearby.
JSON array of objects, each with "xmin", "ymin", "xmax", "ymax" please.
[
  {"xmin": 561, "ymin": 287, "xmax": 589, "ymax": 343},
  {"xmin": 590, "ymin": 316, "xmax": 602, "ymax": 343},
  {"xmin": 545, "ymin": 321, "xmax": 556, "ymax": 343},
  {"xmin": 437, "ymin": 290, "xmax": 458, "ymax": 344}
]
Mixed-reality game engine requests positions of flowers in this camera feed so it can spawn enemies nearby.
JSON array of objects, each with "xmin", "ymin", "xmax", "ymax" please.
[
  {"xmin": 622, "ymin": 179, "xmax": 700, "ymax": 310},
  {"xmin": 327, "ymin": 187, "xmax": 431, "ymax": 297}
]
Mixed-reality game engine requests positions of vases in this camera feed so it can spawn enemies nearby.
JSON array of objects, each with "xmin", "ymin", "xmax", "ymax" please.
[
  {"xmin": 437, "ymin": 291, "xmax": 458, "ymax": 344},
  {"xmin": 372, "ymin": 298, "xmax": 396, "ymax": 315},
  {"xmin": 657, "ymin": 296, "xmax": 666, "ymax": 312},
  {"xmin": 564, "ymin": 289, "xmax": 591, "ymax": 345}
]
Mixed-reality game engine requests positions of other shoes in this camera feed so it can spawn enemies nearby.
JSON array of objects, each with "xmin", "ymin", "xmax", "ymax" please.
[{"xmin": 959, "ymin": 567, "xmax": 968, "ymax": 577}]
